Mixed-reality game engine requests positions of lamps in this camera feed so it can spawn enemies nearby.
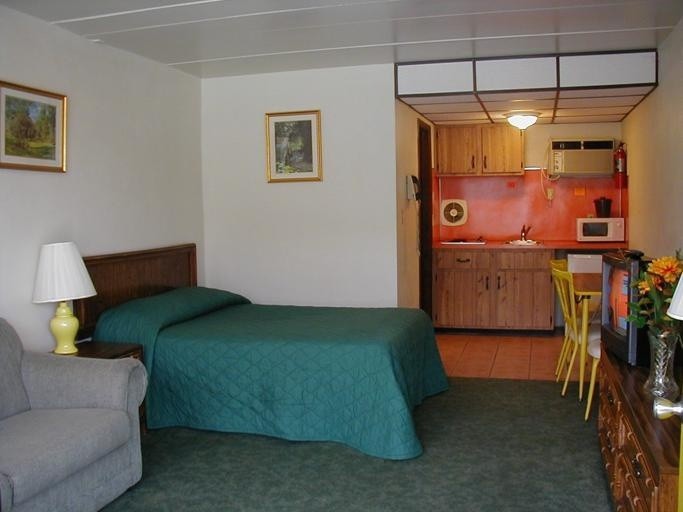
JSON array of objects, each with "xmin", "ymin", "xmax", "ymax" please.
[
  {"xmin": 665, "ymin": 270, "xmax": 683, "ymax": 322},
  {"xmin": 29, "ymin": 241, "xmax": 98, "ymax": 355},
  {"xmin": 506, "ymin": 111, "xmax": 542, "ymax": 137}
]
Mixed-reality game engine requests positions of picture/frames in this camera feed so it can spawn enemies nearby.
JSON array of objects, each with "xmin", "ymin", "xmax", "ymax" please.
[
  {"xmin": 262, "ymin": 109, "xmax": 324, "ymax": 184},
  {"xmin": 0, "ymin": 79, "xmax": 68, "ymax": 174}
]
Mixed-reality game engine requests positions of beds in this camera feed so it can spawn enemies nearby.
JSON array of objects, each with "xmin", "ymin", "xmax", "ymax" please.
[{"xmin": 74, "ymin": 243, "xmax": 450, "ymax": 464}]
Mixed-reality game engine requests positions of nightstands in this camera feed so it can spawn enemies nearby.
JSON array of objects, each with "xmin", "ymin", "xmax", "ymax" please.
[{"xmin": 47, "ymin": 339, "xmax": 150, "ymax": 439}]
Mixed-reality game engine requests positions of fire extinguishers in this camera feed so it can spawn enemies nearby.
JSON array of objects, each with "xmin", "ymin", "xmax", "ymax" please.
[{"xmin": 614, "ymin": 140, "xmax": 628, "ymax": 191}]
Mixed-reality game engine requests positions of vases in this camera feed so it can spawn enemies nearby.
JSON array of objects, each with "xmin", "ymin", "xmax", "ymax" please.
[{"xmin": 642, "ymin": 330, "xmax": 680, "ymax": 404}]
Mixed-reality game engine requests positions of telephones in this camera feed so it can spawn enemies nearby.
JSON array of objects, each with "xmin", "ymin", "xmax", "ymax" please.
[{"xmin": 406, "ymin": 175, "xmax": 423, "ymax": 201}]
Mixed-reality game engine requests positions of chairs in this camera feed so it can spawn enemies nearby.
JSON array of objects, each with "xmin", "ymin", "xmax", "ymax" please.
[
  {"xmin": 544, "ymin": 254, "xmax": 604, "ymax": 422},
  {"xmin": 0, "ymin": 315, "xmax": 150, "ymax": 512}
]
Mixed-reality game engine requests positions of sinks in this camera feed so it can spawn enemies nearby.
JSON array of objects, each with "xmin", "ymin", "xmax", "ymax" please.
[{"xmin": 510, "ymin": 238, "xmax": 541, "ymax": 246}]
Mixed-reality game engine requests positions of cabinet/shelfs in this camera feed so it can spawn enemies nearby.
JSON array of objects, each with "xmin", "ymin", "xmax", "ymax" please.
[
  {"xmin": 433, "ymin": 121, "xmax": 527, "ymax": 176},
  {"xmin": 595, "ymin": 338, "xmax": 682, "ymax": 510},
  {"xmin": 433, "ymin": 248, "xmax": 555, "ymax": 332}
]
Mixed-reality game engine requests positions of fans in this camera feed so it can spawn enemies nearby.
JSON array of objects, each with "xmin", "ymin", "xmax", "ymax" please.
[{"xmin": 440, "ymin": 199, "xmax": 469, "ymax": 228}]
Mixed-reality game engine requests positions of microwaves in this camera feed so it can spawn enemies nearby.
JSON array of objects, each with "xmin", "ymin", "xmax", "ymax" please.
[{"xmin": 575, "ymin": 216, "xmax": 625, "ymax": 243}]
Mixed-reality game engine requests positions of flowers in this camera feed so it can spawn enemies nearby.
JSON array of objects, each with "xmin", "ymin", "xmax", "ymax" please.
[{"xmin": 617, "ymin": 247, "xmax": 683, "ymax": 387}]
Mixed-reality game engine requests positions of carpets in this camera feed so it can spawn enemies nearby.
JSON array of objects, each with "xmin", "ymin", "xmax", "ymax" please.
[{"xmin": 87, "ymin": 376, "xmax": 615, "ymax": 510}]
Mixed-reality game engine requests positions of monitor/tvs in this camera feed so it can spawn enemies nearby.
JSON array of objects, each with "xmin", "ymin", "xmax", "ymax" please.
[{"xmin": 600, "ymin": 250, "xmax": 657, "ymax": 367}]
